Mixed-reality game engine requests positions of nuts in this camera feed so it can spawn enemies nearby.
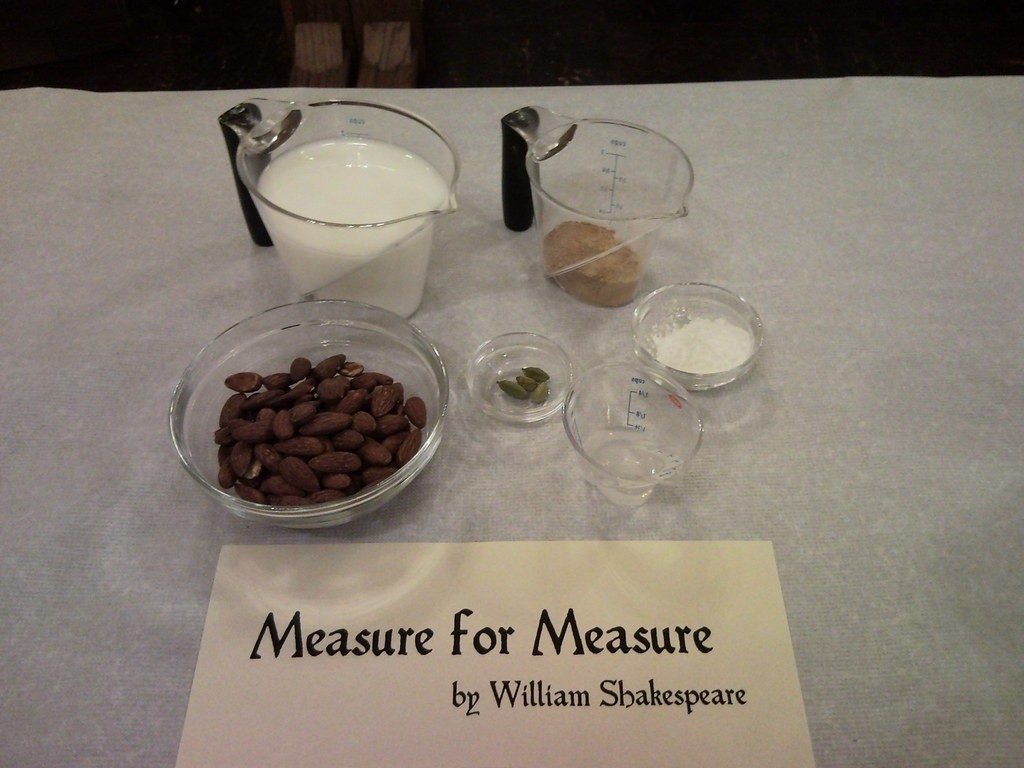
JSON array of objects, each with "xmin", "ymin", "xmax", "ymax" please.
[{"xmin": 213, "ymin": 354, "xmax": 427, "ymax": 508}]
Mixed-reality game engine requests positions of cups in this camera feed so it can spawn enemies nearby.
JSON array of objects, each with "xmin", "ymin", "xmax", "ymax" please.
[{"xmin": 562, "ymin": 361, "xmax": 702, "ymax": 507}]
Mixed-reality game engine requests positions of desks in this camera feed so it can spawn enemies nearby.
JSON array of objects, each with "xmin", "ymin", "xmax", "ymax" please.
[{"xmin": 0, "ymin": 77, "xmax": 1024, "ymax": 768}]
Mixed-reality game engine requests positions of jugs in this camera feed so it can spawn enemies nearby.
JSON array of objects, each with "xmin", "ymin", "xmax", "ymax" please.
[
  {"xmin": 217, "ymin": 97, "xmax": 461, "ymax": 320},
  {"xmin": 500, "ymin": 105, "xmax": 695, "ymax": 309}
]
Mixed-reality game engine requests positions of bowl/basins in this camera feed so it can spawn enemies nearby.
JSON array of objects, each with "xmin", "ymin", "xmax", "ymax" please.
[
  {"xmin": 630, "ymin": 281, "xmax": 764, "ymax": 392},
  {"xmin": 167, "ymin": 298, "xmax": 450, "ymax": 531},
  {"xmin": 465, "ymin": 331, "xmax": 575, "ymax": 424}
]
